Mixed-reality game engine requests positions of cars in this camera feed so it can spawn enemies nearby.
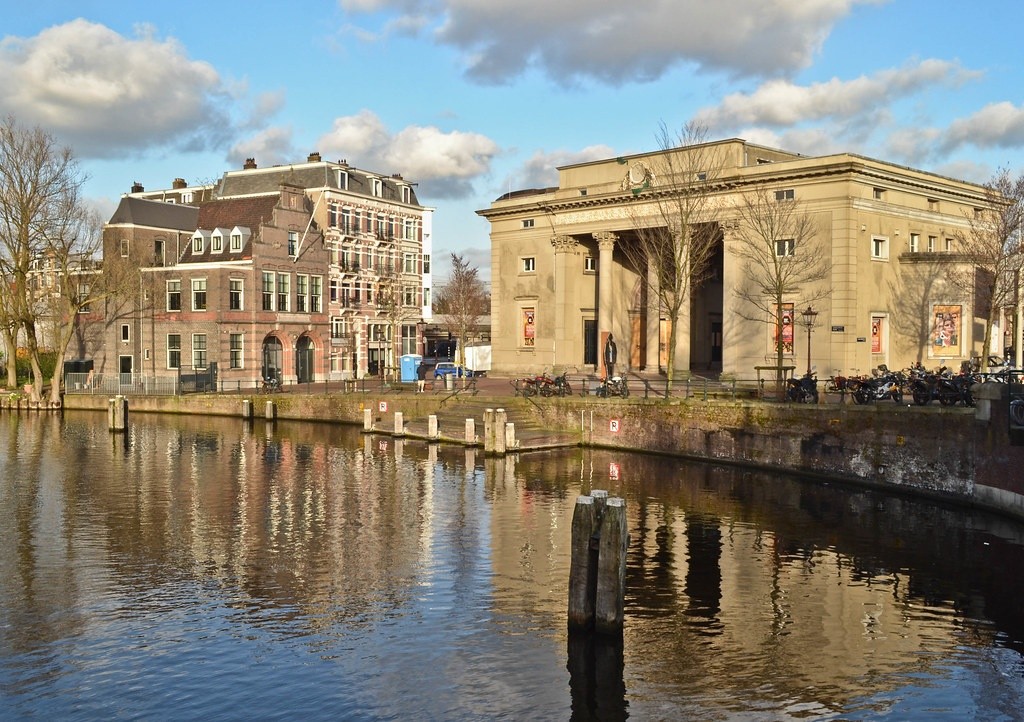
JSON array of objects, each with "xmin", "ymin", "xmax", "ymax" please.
[{"xmin": 433, "ymin": 361, "xmax": 475, "ymax": 381}]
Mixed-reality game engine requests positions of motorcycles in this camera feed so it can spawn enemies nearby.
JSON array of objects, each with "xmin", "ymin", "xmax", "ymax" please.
[
  {"xmin": 785, "ymin": 369, "xmax": 820, "ymax": 404},
  {"xmin": 823, "ymin": 355, "xmax": 1022, "ymax": 408},
  {"xmin": 522, "ymin": 371, "xmax": 572, "ymax": 398},
  {"xmin": 261, "ymin": 371, "xmax": 284, "ymax": 395}
]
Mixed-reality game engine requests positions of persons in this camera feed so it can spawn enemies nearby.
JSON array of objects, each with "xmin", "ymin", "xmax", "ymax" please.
[
  {"xmin": 937, "ymin": 313, "xmax": 957, "ymax": 346},
  {"xmin": 416, "ymin": 362, "xmax": 425, "ymax": 393}
]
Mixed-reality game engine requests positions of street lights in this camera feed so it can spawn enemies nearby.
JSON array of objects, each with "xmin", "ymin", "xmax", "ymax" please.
[{"xmin": 802, "ymin": 304, "xmax": 818, "ymax": 369}]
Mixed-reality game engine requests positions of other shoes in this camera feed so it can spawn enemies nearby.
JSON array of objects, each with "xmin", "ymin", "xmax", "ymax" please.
[{"xmin": 417, "ymin": 390, "xmax": 424, "ymax": 392}]
